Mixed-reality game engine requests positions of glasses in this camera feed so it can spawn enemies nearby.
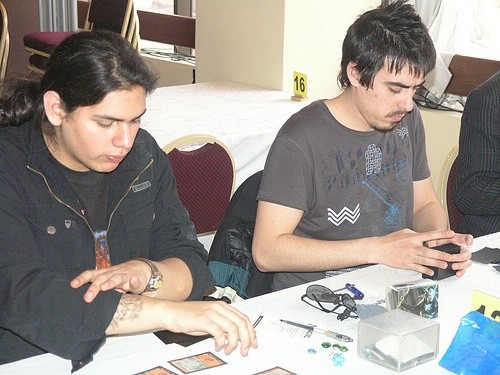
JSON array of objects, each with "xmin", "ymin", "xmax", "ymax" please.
[{"xmin": 301, "ymin": 285, "xmax": 358, "ymax": 320}]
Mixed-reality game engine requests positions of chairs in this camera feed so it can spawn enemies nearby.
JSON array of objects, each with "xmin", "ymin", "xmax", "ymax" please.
[
  {"xmin": 23, "ymin": 0, "xmax": 140, "ymax": 81},
  {"xmin": 160, "ymin": 134, "xmax": 235, "ymax": 235},
  {"xmin": 206, "ymin": 170, "xmax": 271, "ymax": 297}
]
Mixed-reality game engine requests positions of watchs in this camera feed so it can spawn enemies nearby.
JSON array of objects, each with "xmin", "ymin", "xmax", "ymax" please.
[{"xmin": 130, "ymin": 258, "xmax": 163, "ymax": 298}]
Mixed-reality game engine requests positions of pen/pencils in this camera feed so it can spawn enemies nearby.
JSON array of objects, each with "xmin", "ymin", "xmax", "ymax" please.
[{"xmin": 280, "ymin": 319, "xmax": 353, "ymax": 342}]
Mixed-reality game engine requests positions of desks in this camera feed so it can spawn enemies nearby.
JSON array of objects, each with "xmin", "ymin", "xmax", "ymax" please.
[
  {"xmin": 0, "ymin": 231, "xmax": 500, "ymax": 375},
  {"xmin": 137, "ymin": 83, "xmax": 319, "ymax": 195}
]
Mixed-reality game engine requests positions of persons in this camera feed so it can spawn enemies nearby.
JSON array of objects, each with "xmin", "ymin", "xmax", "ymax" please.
[
  {"xmin": 0, "ymin": 30, "xmax": 258, "ymax": 373},
  {"xmin": 251, "ymin": 0, "xmax": 474, "ymax": 278},
  {"xmin": 453, "ymin": 69, "xmax": 500, "ymax": 240}
]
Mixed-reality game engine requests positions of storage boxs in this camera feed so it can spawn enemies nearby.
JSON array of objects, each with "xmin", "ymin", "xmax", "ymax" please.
[{"xmin": 357, "ymin": 310, "xmax": 441, "ymax": 371}]
